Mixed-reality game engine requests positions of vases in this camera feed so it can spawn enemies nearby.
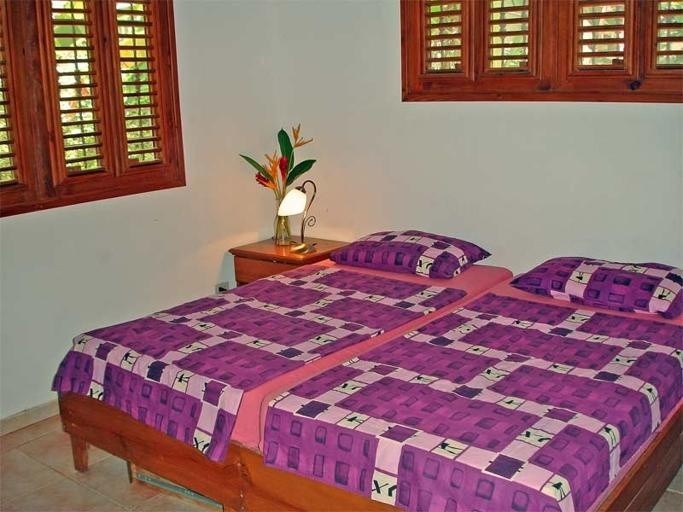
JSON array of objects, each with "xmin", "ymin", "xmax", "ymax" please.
[{"xmin": 273, "ymin": 195, "xmax": 291, "ymax": 245}]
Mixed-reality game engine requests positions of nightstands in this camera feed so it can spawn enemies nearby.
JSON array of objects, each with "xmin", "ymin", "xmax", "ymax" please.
[{"xmin": 228, "ymin": 235, "xmax": 350, "ymax": 287}]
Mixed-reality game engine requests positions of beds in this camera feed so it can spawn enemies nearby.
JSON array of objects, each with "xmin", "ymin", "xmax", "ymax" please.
[{"xmin": 48, "ymin": 261, "xmax": 683, "ymax": 512}]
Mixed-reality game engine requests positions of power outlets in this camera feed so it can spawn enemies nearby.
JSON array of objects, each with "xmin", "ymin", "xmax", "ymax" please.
[{"xmin": 215, "ymin": 282, "xmax": 229, "ymax": 294}]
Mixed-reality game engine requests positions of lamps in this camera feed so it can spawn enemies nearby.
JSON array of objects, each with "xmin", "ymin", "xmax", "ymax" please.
[{"xmin": 277, "ymin": 180, "xmax": 317, "ymax": 255}]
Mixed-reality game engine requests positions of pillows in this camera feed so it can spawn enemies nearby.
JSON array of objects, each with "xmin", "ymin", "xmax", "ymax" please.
[
  {"xmin": 509, "ymin": 256, "xmax": 683, "ymax": 320},
  {"xmin": 329, "ymin": 229, "xmax": 493, "ymax": 280}
]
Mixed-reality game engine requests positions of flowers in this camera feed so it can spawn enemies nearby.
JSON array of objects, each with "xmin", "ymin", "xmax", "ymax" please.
[{"xmin": 237, "ymin": 122, "xmax": 317, "ymax": 240}]
